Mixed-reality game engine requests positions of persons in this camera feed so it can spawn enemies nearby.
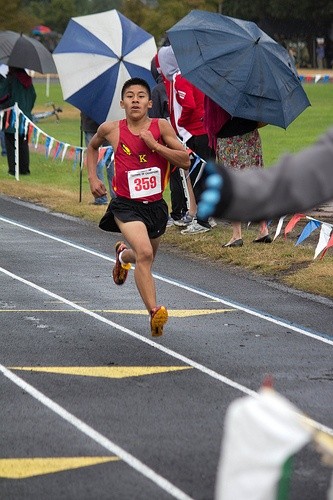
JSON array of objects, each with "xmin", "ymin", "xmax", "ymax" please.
[
  {"xmin": 148, "ymin": 45, "xmax": 217, "ymax": 236},
  {"xmin": 85, "ymin": 77, "xmax": 190, "ymax": 338},
  {"xmin": 204, "ymin": 94, "xmax": 274, "ymax": 248},
  {"xmin": 0, "ymin": 65, "xmax": 37, "ymax": 176},
  {"xmin": 289, "ymin": 44, "xmax": 325, "ymax": 69},
  {"xmin": 195, "ymin": 130, "xmax": 333, "ymax": 229},
  {"xmin": 80, "ymin": 113, "xmax": 118, "ymax": 206},
  {"xmin": 35, "ymin": 34, "xmax": 59, "ymax": 53}
]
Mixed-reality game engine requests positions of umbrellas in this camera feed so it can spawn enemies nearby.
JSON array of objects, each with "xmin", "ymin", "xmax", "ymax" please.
[
  {"xmin": 32, "ymin": 24, "xmax": 51, "ymax": 35},
  {"xmin": 166, "ymin": 9, "xmax": 311, "ymax": 130},
  {"xmin": 51, "ymin": 9, "xmax": 159, "ymax": 125},
  {"xmin": 0, "ymin": 30, "xmax": 58, "ymax": 75}
]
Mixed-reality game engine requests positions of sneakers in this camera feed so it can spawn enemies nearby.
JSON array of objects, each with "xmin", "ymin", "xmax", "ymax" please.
[
  {"xmin": 111, "ymin": 241, "xmax": 128, "ymax": 285},
  {"xmin": 174, "ymin": 213, "xmax": 194, "ymax": 226},
  {"xmin": 149, "ymin": 306, "xmax": 168, "ymax": 336},
  {"xmin": 182, "ymin": 216, "xmax": 216, "ymax": 234}
]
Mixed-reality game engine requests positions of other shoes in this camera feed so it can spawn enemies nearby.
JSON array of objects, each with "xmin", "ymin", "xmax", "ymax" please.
[
  {"xmin": 252, "ymin": 236, "xmax": 272, "ymax": 243},
  {"xmin": 222, "ymin": 239, "xmax": 243, "ymax": 248}
]
